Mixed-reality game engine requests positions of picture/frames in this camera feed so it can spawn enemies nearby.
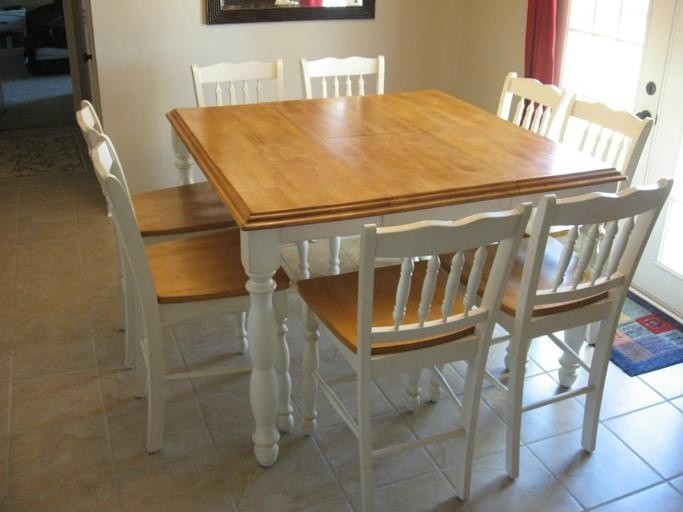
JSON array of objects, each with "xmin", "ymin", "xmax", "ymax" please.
[{"xmin": 206, "ymin": 0, "xmax": 376, "ymax": 24}]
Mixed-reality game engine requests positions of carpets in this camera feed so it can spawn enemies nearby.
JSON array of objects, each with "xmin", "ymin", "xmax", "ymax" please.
[
  {"xmin": 593, "ymin": 295, "xmax": 683, "ymax": 377},
  {"xmin": 0, "ymin": 123, "xmax": 86, "ymax": 180}
]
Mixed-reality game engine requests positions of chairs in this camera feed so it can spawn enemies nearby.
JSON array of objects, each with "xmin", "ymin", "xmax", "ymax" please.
[
  {"xmin": 442, "ymin": 179, "xmax": 673, "ymax": 476},
  {"xmin": 91, "ymin": 138, "xmax": 294, "ymax": 453},
  {"xmin": 193, "ymin": 60, "xmax": 285, "ymax": 107},
  {"xmin": 300, "ymin": 56, "xmax": 385, "ymax": 98},
  {"xmin": 75, "ymin": 101, "xmax": 239, "ymax": 236},
  {"xmin": 498, "ymin": 73, "xmax": 566, "ymax": 139},
  {"xmin": 298, "ymin": 202, "xmax": 531, "ymax": 512},
  {"xmin": 560, "ymin": 94, "xmax": 654, "ymax": 189}
]
